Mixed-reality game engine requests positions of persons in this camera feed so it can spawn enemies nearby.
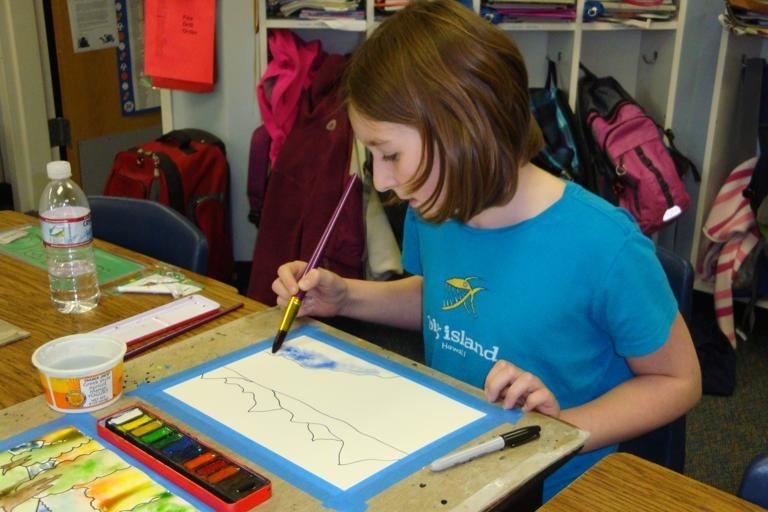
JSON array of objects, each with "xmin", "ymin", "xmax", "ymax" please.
[{"xmin": 271, "ymin": 0, "xmax": 702, "ymax": 454}]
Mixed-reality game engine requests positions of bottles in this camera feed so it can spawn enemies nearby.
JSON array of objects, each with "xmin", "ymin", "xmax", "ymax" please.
[{"xmin": 40, "ymin": 160, "xmax": 101, "ymax": 316}]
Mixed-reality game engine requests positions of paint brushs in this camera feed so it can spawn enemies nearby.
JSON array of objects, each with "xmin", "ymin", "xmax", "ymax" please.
[
  {"xmin": 272, "ymin": 171, "xmax": 359, "ymax": 353},
  {"xmin": 125, "ymin": 301, "xmax": 246, "ymax": 359}
]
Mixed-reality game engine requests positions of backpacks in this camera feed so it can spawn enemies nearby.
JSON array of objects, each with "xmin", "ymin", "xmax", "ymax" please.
[
  {"xmin": 527, "ymin": 61, "xmax": 593, "ymax": 187},
  {"xmin": 576, "ymin": 60, "xmax": 702, "ymax": 236}
]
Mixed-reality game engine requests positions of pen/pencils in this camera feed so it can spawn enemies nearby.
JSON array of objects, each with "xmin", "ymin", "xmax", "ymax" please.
[{"xmin": 435, "ymin": 424, "xmax": 544, "ymax": 470}]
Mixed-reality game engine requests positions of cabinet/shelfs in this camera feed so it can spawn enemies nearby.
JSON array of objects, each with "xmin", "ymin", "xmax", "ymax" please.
[
  {"xmin": 253, "ymin": 0, "xmax": 688, "ymax": 248},
  {"xmin": 688, "ymin": 0, "xmax": 768, "ymax": 311}
]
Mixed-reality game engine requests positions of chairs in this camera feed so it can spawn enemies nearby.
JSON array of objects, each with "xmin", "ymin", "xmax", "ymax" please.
[
  {"xmin": 85, "ymin": 195, "xmax": 208, "ymax": 277},
  {"xmin": 620, "ymin": 243, "xmax": 694, "ymax": 473},
  {"xmin": 735, "ymin": 449, "xmax": 768, "ymax": 511}
]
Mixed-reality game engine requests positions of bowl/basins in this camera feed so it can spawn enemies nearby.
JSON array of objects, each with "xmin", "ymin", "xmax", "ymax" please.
[{"xmin": 32, "ymin": 332, "xmax": 128, "ymax": 412}]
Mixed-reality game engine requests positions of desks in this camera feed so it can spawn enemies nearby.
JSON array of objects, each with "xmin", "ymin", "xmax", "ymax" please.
[
  {"xmin": 533, "ymin": 450, "xmax": 768, "ymax": 512},
  {"xmin": 0, "ymin": 208, "xmax": 592, "ymax": 511}
]
